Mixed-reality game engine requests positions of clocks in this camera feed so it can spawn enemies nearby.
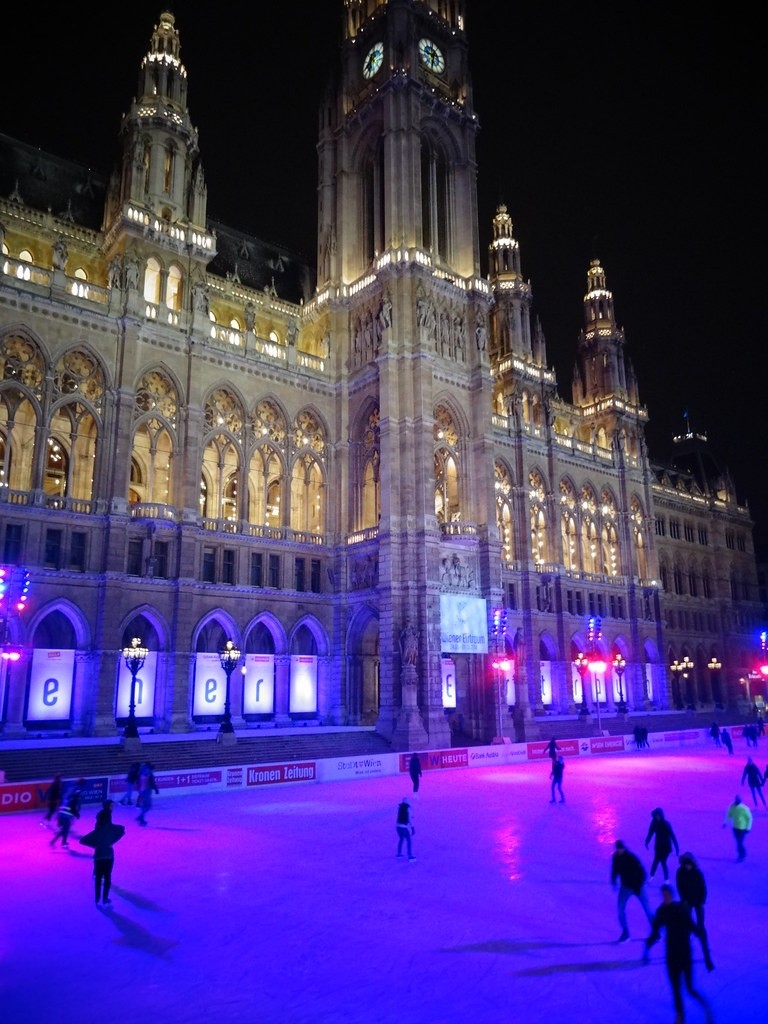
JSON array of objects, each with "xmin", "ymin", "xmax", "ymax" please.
[
  {"xmin": 418, "ymin": 37, "xmax": 446, "ymax": 74},
  {"xmin": 363, "ymin": 43, "xmax": 384, "ymax": 80}
]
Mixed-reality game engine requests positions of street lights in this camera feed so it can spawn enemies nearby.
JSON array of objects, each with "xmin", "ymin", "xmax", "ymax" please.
[
  {"xmin": 493, "ymin": 653, "xmax": 514, "ymax": 739},
  {"xmin": 613, "ymin": 654, "xmax": 630, "ymax": 713},
  {"xmin": 217, "ymin": 638, "xmax": 241, "ymax": 732},
  {"xmin": 670, "ymin": 654, "xmax": 694, "ymax": 709},
  {"xmin": 122, "ymin": 638, "xmax": 148, "ymax": 737},
  {"xmin": 708, "ymin": 656, "xmax": 723, "ymax": 713},
  {"xmin": 575, "ymin": 654, "xmax": 591, "ymax": 718}
]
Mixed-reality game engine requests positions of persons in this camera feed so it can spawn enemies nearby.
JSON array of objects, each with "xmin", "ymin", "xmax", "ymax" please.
[
  {"xmin": 633, "ymin": 725, "xmax": 651, "ymax": 750},
  {"xmin": 542, "ymin": 736, "xmax": 561, "ymax": 766},
  {"xmin": 398, "ymin": 623, "xmax": 420, "ymax": 665},
  {"xmin": 513, "ymin": 391, "xmax": 647, "ymax": 457},
  {"xmin": 219, "ymin": 710, "xmax": 236, "ymax": 733},
  {"xmin": 722, "ymin": 793, "xmax": 752, "ymax": 862},
  {"xmin": 80, "ymin": 798, "xmax": 125, "ymax": 905},
  {"xmin": 646, "ymin": 880, "xmax": 706, "ymax": 1024},
  {"xmin": 243, "ymin": 301, "xmax": 299, "ymax": 345},
  {"xmin": 120, "ymin": 759, "xmax": 160, "ymax": 827},
  {"xmin": 42, "ymin": 772, "xmax": 86, "ymax": 850},
  {"xmin": 409, "ymin": 752, "xmax": 422, "ymax": 793},
  {"xmin": 610, "ymin": 838, "xmax": 655, "ymax": 943},
  {"xmin": 741, "ymin": 756, "xmax": 768, "ymax": 809},
  {"xmin": 106, "ymin": 249, "xmax": 140, "ymax": 291},
  {"xmin": 51, "ymin": 235, "xmax": 68, "ymax": 270},
  {"xmin": 548, "ymin": 755, "xmax": 565, "ymax": 803},
  {"xmin": 645, "ymin": 807, "xmax": 679, "ymax": 885},
  {"xmin": 123, "ymin": 717, "xmax": 138, "ymax": 738},
  {"xmin": 742, "ymin": 717, "xmax": 765, "ymax": 748},
  {"xmin": 350, "ymin": 552, "xmax": 376, "ymax": 589},
  {"xmin": 676, "ymin": 852, "xmax": 713, "ymax": 970},
  {"xmin": 192, "ymin": 280, "xmax": 208, "ymax": 314},
  {"xmin": 442, "ymin": 557, "xmax": 475, "ymax": 588},
  {"xmin": 395, "ymin": 796, "xmax": 417, "ymax": 863},
  {"xmin": 354, "ymin": 297, "xmax": 489, "ymax": 356},
  {"xmin": 709, "ymin": 722, "xmax": 734, "ymax": 756}
]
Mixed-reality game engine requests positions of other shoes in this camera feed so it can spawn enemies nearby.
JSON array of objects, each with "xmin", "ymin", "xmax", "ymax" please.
[
  {"xmin": 705, "ymin": 958, "xmax": 714, "ymax": 973},
  {"xmin": 103, "ymin": 899, "xmax": 113, "ymax": 905},
  {"xmin": 559, "ymin": 799, "xmax": 565, "ymax": 804},
  {"xmin": 736, "ymin": 855, "xmax": 745, "ymax": 862},
  {"xmin": 47, "ymin": 844, "xmax": 58, "ymax": 850},
  {"xmin": 412, "ymin": 790, "xmax": 419, "ymax": 800},
  {"xmin": 136, "ymin": 816, "xmax": 148, "ymax": 827},
  {"xmin": 127, "ymin": 799, "xmax": 135, "ymax": 805},
  {"xmin": 39, "ymin": 821, "xmax": 50, "ymax": 829},
  {"xmin": 408, "ymin": 857, "xmax": 416, "ymax": 861},
  {"xmin": 396, "ymin": 855, "xmax": 404, "ymax": 859},
  {"xmin": 61, "ymin": 843, "xmax": 70, "ymax": 848},
  {"xmin": 648, "ymin": 874, "xmax": 653, "ymax": 882},
  {"xmin": 118, "ymin": 799, "xmax": 125, "ymax": 808},
  {"xmin": 550, "ymin": 799, "xmax": 556, "ymax": 803},
  {"xmin": 618, "ymin": 930, "xmax": 631, "ymax": 944},
  {"xmin": 94, "ymin": 898, "xmax": 101, "ymax": 904}
]
